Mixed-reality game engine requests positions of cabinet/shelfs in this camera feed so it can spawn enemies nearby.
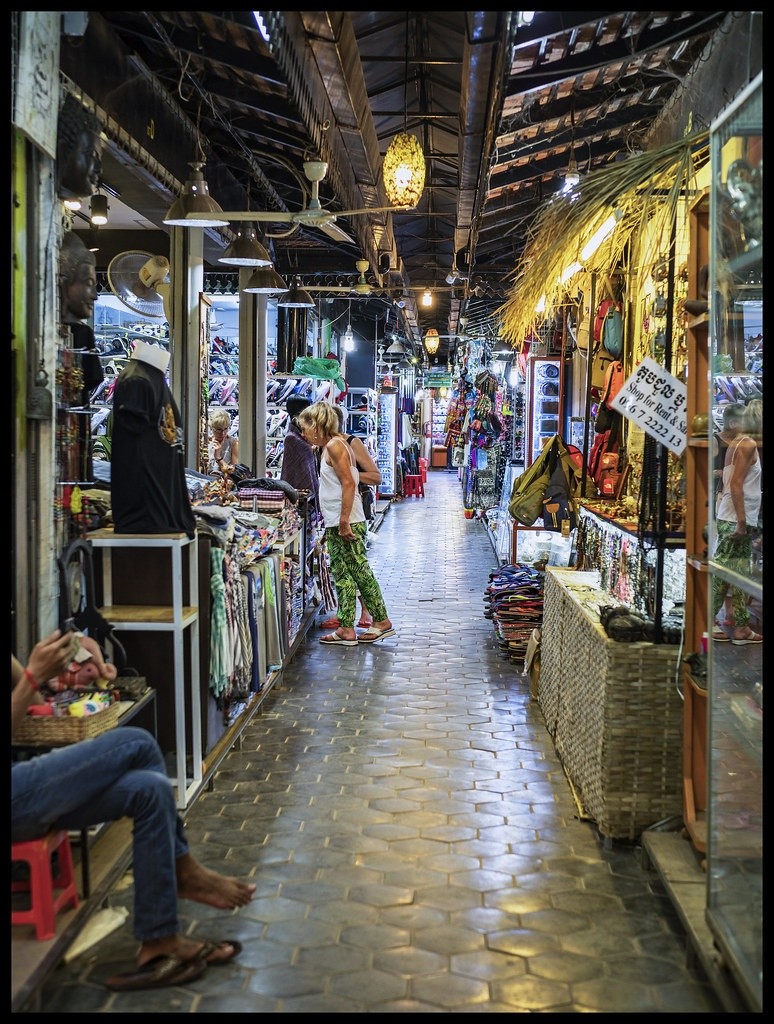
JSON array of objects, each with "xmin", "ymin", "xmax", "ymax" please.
[
  {"xmin": 11, "ymin": 689, "xmax": 158, "ymax": 882},
  {"xmin": 524, "ymin": 357, "xmax": 561, "ymax": 473},
  {"xmin": 86, "ymin": 530, "xmax": 203, "ymax": 810},
  {"xmin": 431, "ymin": 398, "xmax": 448, "ymax": 466},
  {"xmin": 412, "ymin": 402, "xmax": 425, "ymax": 457},
  {"xmin": 91, "ymin": 302, "xmax": 335, "ymax": 479},
  {"xmin": 346, "ymin": 388, "xmax": 378, "ymax": 512},
  {"xmin": 679, "ymin": 72, "xmax": 763, "ymax": 1011}
]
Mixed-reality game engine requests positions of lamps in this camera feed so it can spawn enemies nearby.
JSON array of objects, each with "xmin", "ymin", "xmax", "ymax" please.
[
  {"xmin": 492, "ymin": 341, "xmax": 513, "ymax": 362},
  {"xmin": 242, "ymin": 250, "xmax": 289, "ymax": 293},
  {"xmin": 217, "ymin": 183, "xmax": 273, "ymax": 266},
  {"xmin": 395, "ymin": 359, "xmax": 412, "ymax": 369},
  {"xmin": 386, "ymin": 335, "xmax": 408, "ymax": 353},
  {"xmin": 89, "ymin": 184, "xmax": 110, "ymax": 225},
  {"xmin": 162, "ymin": 103, "xmax": 230, "ymax": 227},
  {"xmin": 426, "ymin": 329, "xmax": 439, "ymax": 353},
  {"xmin": 277, "ymin": 276, "xmax": 316, "ymax": 308},
  {"xmin": 383, "ymin": 13, "xmax": 426, "ymax": 207}
]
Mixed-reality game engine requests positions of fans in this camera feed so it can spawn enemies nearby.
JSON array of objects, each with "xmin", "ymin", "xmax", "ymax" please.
[
  {"xmin": 185, "ymin": 147, "xmax": 413, "ymax": 244},
  {"xmin": 377, "ymin": 363, "xmax": 401, "ymax": 377},
  {"xmin": 107, "ymin": 250, "xmax": 170, "ymax": 323},
  {"xmin": 440, "ymin": 314, "xmax": 486, "ymax": 341},
  {"xmin": 375, "ymin": 346, "xmax": 399, "ymax": 366},
  {"xmin": 297, "ymin": 255, "xmax": 465, "ymax": 294}
]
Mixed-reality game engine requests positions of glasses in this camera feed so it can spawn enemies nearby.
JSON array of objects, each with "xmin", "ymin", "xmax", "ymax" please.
[
  {"xmin": 212, "ymin": 425, "xmax": 231, "ymax": 433},
  {"xmin": 492, "ymin": 391, "xmax": 526, "ymax": 495}
]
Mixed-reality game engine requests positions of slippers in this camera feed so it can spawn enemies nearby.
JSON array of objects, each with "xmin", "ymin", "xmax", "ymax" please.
[
  {"xmin": 104, "ymin": 952, "xmax": 207, "ymax": 991},
  {"xmin": 732, "ymin": 631, "xmax": 763, "ymax": 645},
  {"xmin": 356, "ymin": 618, "xmax": 372, "ymax": 628},
  {"xmin": 711, "ymin": 625, "xmax": 731, "ymax": 642},
  {"xmin": 481, "ymin": 561, "xmax": 545, "ymax": 663},
  {"xmin": 319, "ymin": 618, "xmax": 340, "ymax": 628},
  {"xmin": 723, "ymin": 619, "xmax": 738, "ymax": 628},
  {"xmin": 318, "ymin": 632, "xmax": 358, "ymax": 645},
  {"xmin": 357, "ymin": 626, "xmax": 396, "ymax": 643},
  {"xmin": 183, "ymin": 936, "xmax": 242, "ymax": 966}
]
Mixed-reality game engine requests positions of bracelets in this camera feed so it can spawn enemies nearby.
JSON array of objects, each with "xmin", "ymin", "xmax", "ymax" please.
[
  {"xmin": 217, "ymin": 458, "xmax": 223, "ymax": 462},
  {"xmin": 23, "ymin": 668, "xmax": 40, "ymax": 692}
]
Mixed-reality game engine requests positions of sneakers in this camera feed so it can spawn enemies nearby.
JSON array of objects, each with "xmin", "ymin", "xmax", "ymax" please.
[{"xmin": 208, "ymin": 335, "xmax": 378, "ymax": 479}]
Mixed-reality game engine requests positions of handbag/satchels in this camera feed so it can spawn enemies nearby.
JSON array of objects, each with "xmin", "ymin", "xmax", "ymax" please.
[
  {"xmin": 541, "ymin": 436, "xmax": 577, "ymax": 533},
  {"xmin": 508, "ymin": 434, "xmax": 556, "ymax": 526},
  {"xmin": 555, "ymin": 434, "xmax": 597, "ymax": 510},
  {"xmin": 565, "ymin": 287, "xmax": 627, "ymax": 500}
]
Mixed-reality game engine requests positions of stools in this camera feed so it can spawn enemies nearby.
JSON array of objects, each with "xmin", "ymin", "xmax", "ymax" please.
[
  {"xmin": 405, "ymin": 475, "xmax": 424, "ymax": 498},
  {"xmin": 12, "ymin": 829, "xmax": 80, "ymax": 942}
]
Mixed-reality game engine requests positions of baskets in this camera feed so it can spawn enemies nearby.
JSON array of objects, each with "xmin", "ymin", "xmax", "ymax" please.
[{"xmin": 12, "ymin": 701, "xmax": 119, "ymax": 744}]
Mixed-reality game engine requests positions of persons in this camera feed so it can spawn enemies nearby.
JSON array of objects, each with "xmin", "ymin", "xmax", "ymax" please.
[
  {"xmin": 59, "ymin": 231, "xmax": 104, "ymax": 485},
  {"xmin": 205, "ymin": 408, "xmax": 239, "ymax": 475},
  {"xmin": 111, "ymin": 341, "xmax": 198, "ymax": 539},
  {"xmin": 12, "ymin": 630, "xmax": 258, "ymax": 968},
  {"xmin": 280, "ymin": 396, "xmax": 319, "ymax": 494},
  {"xmin": 712, "ymin": 399, "xmax": 765, "ymax": 644},
  {"xmin": 294, "ymin": 401, "xmax": 395, "ymax": 648}
]
